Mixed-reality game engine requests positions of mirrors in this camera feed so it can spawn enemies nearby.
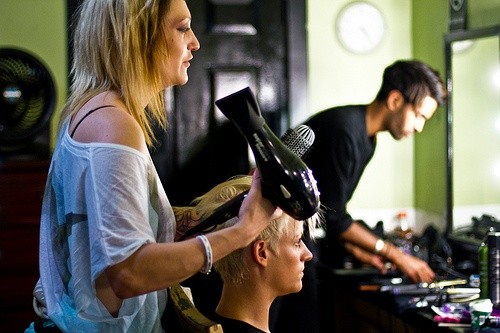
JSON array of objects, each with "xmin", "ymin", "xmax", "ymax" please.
[{"xmin": 442, "ymin": 25, "xmax": 500, "ymax": 234}]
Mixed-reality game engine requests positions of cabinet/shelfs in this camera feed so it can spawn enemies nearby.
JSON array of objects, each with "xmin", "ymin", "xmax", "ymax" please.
[{"xmin": 0, "ymin": 160, "xmax": 50, "ymax": 333}]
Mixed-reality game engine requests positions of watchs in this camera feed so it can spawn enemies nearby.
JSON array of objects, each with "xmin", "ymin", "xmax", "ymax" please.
[{"xmin": 373, "ymin": 238, "xmax": 385, "ymax": 256}]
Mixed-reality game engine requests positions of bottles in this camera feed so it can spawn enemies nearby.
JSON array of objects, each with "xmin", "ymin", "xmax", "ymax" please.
[
  {"xmin": 469, "ymin": 299, "xmax": 492, "ymax": 333},
  {"xmin": 487, "ymin": 233, "xmax": 500, "ymax": 306},
  {"xmin": 393, "ymin": 212, "xmax": 413, "ymax": 249},
  {"xmin": 479, "ymin": 226, "xmax": 494, "ymax": 299}
]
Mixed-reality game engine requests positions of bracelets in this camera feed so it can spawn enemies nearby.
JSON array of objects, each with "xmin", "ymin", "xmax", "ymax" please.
[
  {"xmin": 195, "ymin": 234, "xmax": 213, "ymax": 275},
  {"xmin": 383, "ymin": 243, "xmax": 392, "ymax": 261}
]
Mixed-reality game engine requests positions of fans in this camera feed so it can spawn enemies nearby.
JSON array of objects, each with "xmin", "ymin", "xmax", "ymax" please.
[{"xmin": 0, "ymin": 46, "xmax": 57, "ymax": 160}]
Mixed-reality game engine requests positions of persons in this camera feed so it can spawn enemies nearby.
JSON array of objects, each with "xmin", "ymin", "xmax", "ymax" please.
[
  {"xmin": 24, "ymin": 0, "xmax": 277, "ymax": 333},
  {"xmin": 171, "ymin": 174, "xmax": 326, "ymax": 333},
  {"xmin": 284, "ymin": 60, "xmax": 446, "ymax": 333}
]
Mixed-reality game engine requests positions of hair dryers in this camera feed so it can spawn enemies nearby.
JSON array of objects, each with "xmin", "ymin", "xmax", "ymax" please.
[
  {"xmin": 172, "ymin": 86, "xmax": 322, "ymax": 242},
  {"xmin": 409, "ymin": 224, "xmax": 452, "ymax": 259}
]
